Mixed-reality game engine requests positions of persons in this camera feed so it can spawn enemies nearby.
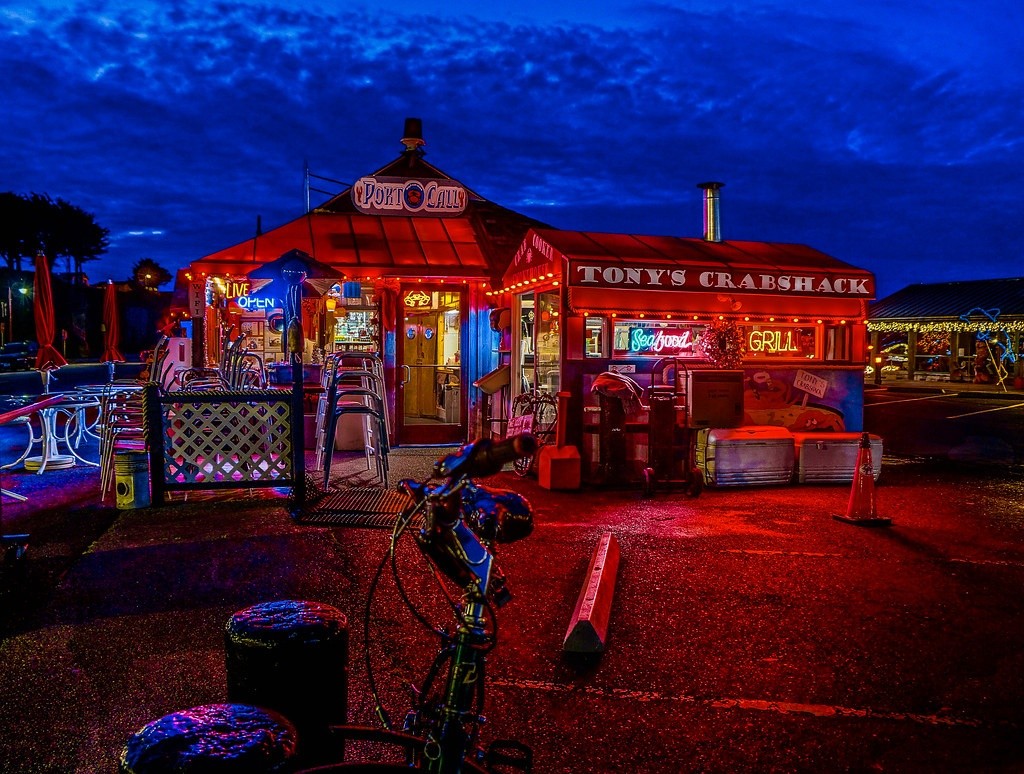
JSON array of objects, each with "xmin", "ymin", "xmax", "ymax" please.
[{"xmin": 60, "ymin": 327, "xmax": 90, "ymax": 362}]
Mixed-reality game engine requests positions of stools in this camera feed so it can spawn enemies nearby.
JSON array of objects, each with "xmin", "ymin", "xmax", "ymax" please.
[{"xmin": 314, "ymin": 349, "xmax": 394, "ymax": 491}]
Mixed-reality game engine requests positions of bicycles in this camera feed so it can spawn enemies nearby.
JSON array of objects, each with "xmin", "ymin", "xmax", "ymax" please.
[{"xmin": 289, "ymin": 431, "xmax": 538, "ymax": 774}]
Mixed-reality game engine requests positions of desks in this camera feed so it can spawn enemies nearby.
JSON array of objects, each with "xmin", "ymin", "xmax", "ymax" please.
[{"xmin": 10, "ymin": 381, "xmax": 123, "ymax": 473}]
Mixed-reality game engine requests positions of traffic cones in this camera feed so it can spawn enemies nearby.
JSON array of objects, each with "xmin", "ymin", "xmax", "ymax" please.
[{"xmin": 832, "ymin": 431, "xmax": 892, "ymax": 526}]
